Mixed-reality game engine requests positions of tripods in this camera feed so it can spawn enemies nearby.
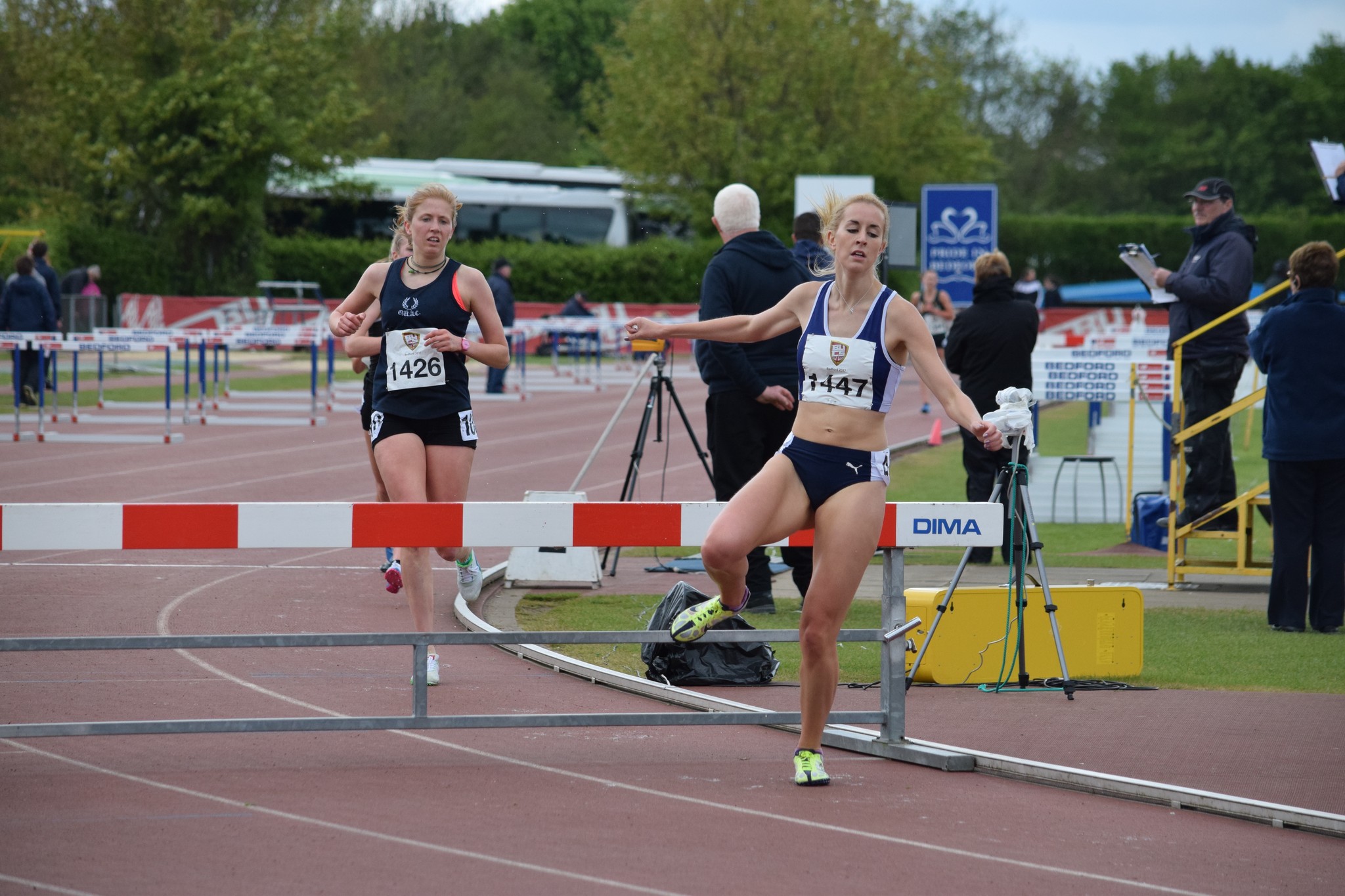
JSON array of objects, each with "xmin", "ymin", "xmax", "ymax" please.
[
  {"xmin": 902, "ymin": 447, "xmax": 1074, "ymax": 701},
  {"xmin": 602, "ymin": 368, "xmax": 713, "ymax": 576}
]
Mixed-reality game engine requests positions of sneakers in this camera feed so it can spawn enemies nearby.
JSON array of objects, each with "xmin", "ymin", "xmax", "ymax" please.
[
  {"xmin": 385, "ymin": 559, "xmax": 403, "ymax": 594},
  {"xmin": 793, "ymin": 748, "xmax": 830, "ymax": 786},
  {"xmin": 410, "ymin": 651, "xmax": 439, "ymax": 686},
  {"xmin": 455, "ymin": 547, "xmax": 483, "ymax": 603},
  {"xmin": 669, "ymin": 585, "xmax": 751, "ymax": 644}
]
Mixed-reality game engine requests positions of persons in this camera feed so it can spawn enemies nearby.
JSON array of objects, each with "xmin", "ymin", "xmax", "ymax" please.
[
  {"xmin": 328, "ymin": 182, "xmax": 516, "ymax": 686},
  {"xmin": 0, "ymin": 239, "xmax": 106, "ymax": 408},
  {"xmin": 624, "ymin": 175, "xmax": 1344, "ymax": 784}
]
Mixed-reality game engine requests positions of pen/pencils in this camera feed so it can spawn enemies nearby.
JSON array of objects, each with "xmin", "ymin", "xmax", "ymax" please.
[{"xmin": 1153, "ymin": 253, "xmax": 1161, "ymax": 259}]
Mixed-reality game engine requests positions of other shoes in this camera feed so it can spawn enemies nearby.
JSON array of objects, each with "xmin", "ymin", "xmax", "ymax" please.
[
  {"xmin": 1156, "ymin": 516, "xmax": 1184, "ymax": 527},
  {"xmin": 43, "ymin": 377, "xmax": 54, "ymax": 390},
  {"xmin": 743, "ymin": 595, "xmax": 778, "ymax": 614},
  {"xmin": 380, "ymin": 562, "xmax": 392, "ymax": 573},
  {"xmin": 1272, "ymin": 622, "xmax": 1339, "ymax": 633},
  {"xmin": 921, "ymin": 402, "xmax": 931, "ymax": 413},
  {"xmin": 23, "ymin": 383, "xmax": 38, "ymax": 406}
]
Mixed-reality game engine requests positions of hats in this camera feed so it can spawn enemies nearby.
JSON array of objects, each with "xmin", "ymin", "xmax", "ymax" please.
[{"xmin": 1183, "ymin": 178, "xmax": 1235, "ymax": 207}]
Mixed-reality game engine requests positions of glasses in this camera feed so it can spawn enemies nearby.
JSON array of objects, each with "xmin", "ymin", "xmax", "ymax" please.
[{"xmin": 1187, "ymin": 198, "xmax": 1225, "ymax": 205}]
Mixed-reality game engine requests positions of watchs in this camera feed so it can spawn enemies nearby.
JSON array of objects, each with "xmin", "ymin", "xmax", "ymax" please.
[{"xmin": 460, "ymin": 337, "xmax": 470, "ymax": 353}]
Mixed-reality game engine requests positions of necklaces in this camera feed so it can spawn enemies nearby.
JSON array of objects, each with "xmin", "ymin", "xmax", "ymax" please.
[
  {"xmin": 407, "ymin": 255, "xmax": 446, "ymax": 274},
  {"xmin": 835, "ymin": 277, "xmax": 875, "ymax": 314},
  {"xmin": 411, "ymin": 255, "xmax": 446, "ymax": 268}
]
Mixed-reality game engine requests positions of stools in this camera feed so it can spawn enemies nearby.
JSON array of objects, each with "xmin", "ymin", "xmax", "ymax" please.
[{"xmin": 1051, "ymin": 455, "xmax": 1124, "ymax": 524}]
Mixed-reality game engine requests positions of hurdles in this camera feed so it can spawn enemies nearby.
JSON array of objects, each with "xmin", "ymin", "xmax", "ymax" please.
[
  {"xmin": 2, "ymin": 501, "xmax": 1004, "ymax": 772},
  {"xmin": 0, "ymin": 327, "xmax": 370, "ymax": 444},
  {"xmin": 1022, "ymin": 324, "xmax": 1175, "ymax": 524},
  {"xmin": 465, "ymin": 316, "xmax": 702, "ymax": 401}
]
